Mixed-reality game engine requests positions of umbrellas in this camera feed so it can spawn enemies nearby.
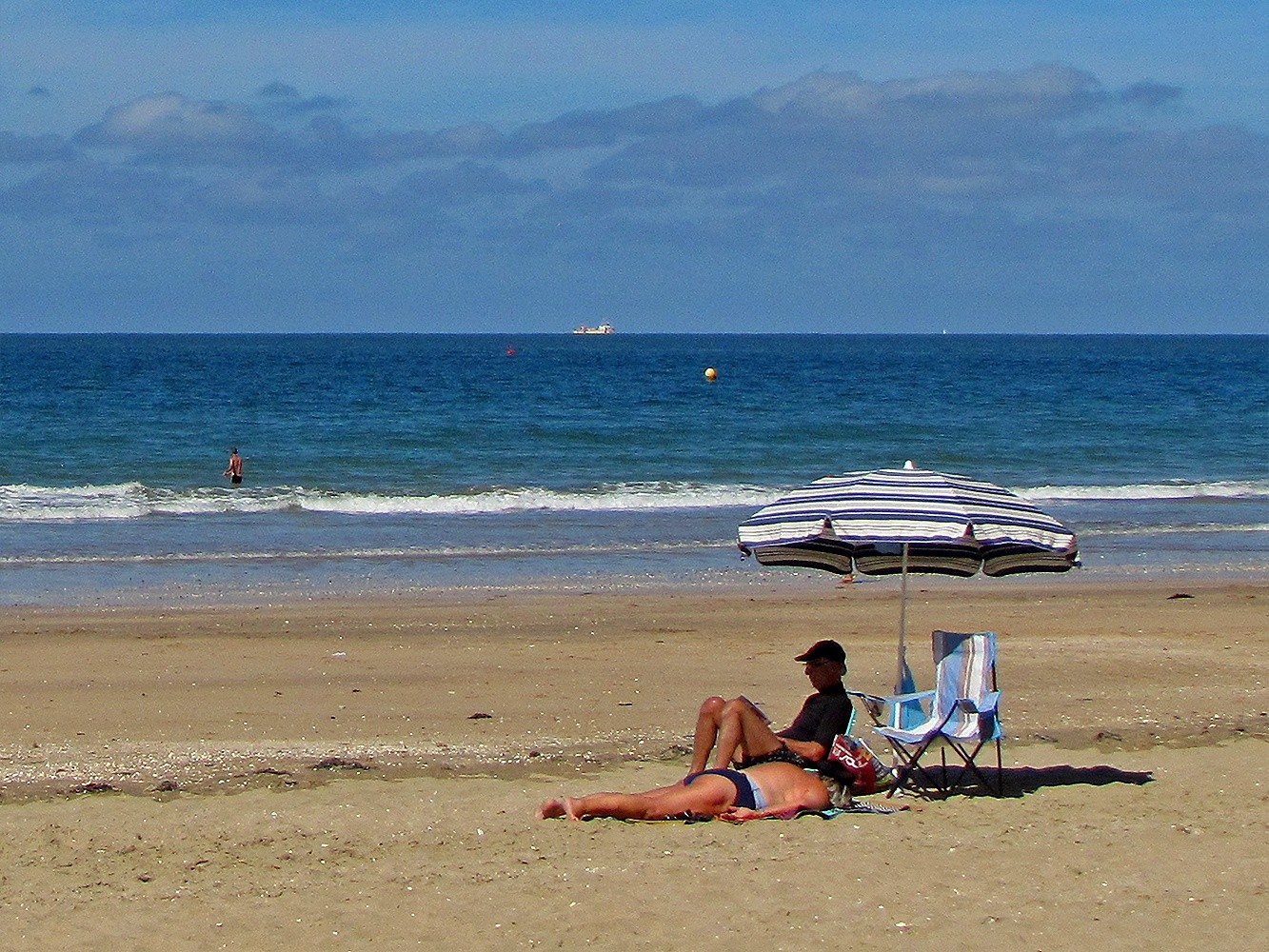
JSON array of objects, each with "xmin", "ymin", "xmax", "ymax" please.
[{"xmin": 736, "ymin": 460, "xmax": 1081, "ymax": 795}]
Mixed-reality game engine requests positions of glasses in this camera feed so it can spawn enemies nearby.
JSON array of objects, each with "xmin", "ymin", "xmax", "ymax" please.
[{"xmin": 802, "ymin": 660, "xmax": 830, "ymax": 672}]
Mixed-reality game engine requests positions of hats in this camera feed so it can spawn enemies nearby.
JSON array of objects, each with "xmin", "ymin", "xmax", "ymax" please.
[{"xmin": 794, "ymin": 641, "xmax": 846, "ymax": 665}]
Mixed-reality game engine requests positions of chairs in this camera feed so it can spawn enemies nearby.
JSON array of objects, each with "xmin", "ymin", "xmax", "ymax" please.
[{"xmin": 803, "ymin": 630, "xmax": 1002, "ymax": 801}]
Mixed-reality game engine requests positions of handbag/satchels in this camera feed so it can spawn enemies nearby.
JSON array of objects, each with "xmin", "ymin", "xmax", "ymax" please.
[
  {"xmin": 850, "ymin": 737, "xmax": 895, "ymax": 789},
  {"xmin": 830, "ymin": 734, "xmax": 876, "ymax": 793}
]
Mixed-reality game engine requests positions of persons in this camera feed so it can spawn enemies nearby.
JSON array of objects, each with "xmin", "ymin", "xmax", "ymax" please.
[
  {"xmin": 224, "ymin": 447, "xmax": 242, "ymax": 484},
  {"xmin": 843, "ymin": 574, "xmax": 853, "ymax": 582},
  {"xmin": 536, "ymin": 761, "xmax": 852, "ymax": 823},
  {"xmin": 688, "ymin": 640, "xmax": 852, "ymax": 775}
]
